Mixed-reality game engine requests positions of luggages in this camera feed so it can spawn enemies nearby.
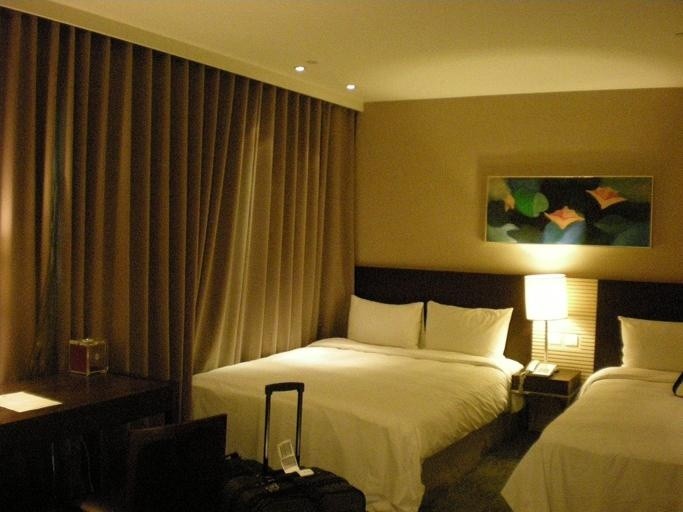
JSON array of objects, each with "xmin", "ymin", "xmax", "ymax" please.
[{"xmin": 188, "ymin": 383, "xmax": 367, "ymax": 512}]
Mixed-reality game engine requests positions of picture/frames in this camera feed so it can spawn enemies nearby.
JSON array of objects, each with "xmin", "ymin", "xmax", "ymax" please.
[{"xmin": 483, "ymin": 175, "xmax": 654, "ymax": 249}]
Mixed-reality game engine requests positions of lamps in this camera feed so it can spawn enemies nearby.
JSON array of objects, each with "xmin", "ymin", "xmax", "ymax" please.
[{"xmin": 522, "ymin": 271, "xmax": 566, "ymax": 373}]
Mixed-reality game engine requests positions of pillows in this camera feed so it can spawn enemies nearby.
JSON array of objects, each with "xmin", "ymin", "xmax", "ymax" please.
[
  {"xmin": 616, "ymin": 315, "xmax": 682, "ymax": 372},
  {"xmin": 343, "ymin": 294, "xmax": 512, "ymax": 361}
]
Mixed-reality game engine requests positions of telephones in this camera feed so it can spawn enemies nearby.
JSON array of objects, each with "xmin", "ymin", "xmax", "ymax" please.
[{"xmin": 524, "ymin": 360, "xmax": 557, "ymax": 377}]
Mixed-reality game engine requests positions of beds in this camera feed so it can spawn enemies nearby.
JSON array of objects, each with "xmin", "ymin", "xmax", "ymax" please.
[
  {"xmin": 538, "ymin": 277, "xmax": 681, "ymax": 511},
  {"xmin": 190, "ymin": 261, "xmax": 530, "ymax": 510}
]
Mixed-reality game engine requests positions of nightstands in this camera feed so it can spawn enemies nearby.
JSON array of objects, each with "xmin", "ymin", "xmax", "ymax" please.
[{"xmin": 510, "ymin": 367, "xmax": 581, "ymax": 436}]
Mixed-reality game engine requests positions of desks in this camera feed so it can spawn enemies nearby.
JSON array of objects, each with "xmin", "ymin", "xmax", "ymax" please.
[{"xmin": 0, "ymin": 368, "xmax": 180, "ymax": 509}]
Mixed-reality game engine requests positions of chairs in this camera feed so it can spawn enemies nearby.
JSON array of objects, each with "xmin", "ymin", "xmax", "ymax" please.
[{"xmin": 76, "ymin": 413, "xmax": 227, "ymax": 510}]
